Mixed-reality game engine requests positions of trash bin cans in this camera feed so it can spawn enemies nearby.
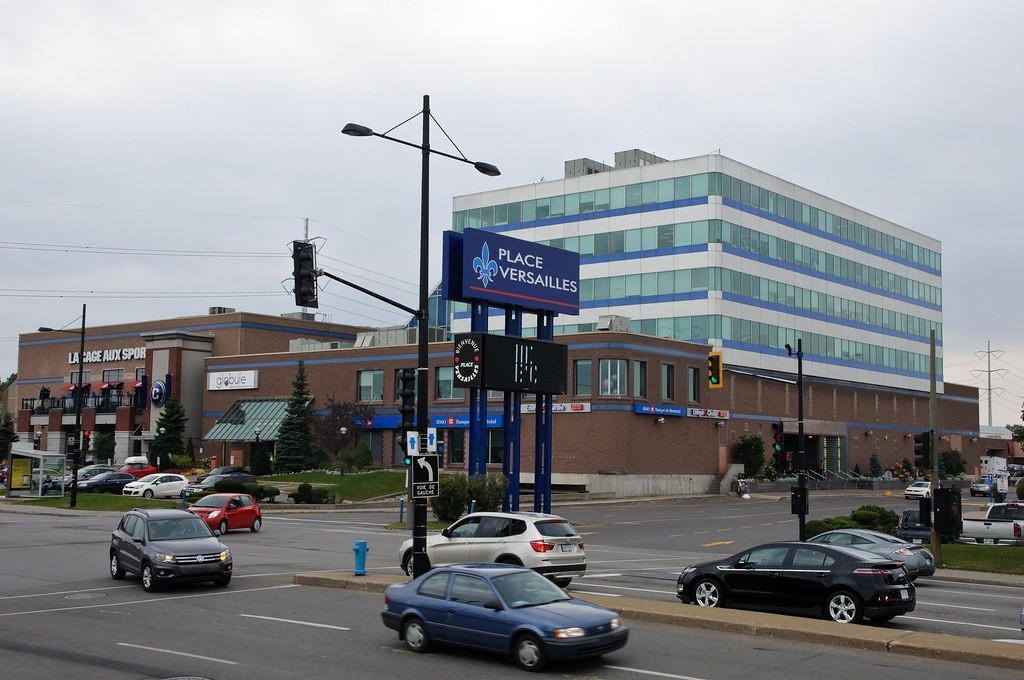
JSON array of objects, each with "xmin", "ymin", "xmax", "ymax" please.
[
  {"xmin": 738, "ymin": 473, "xmax": 750, "ymax": 496},
  {"xmin": 791, "ymin": 486, "xmax": 809, "ymax": 514},
  {"xmin": 934, "ymin": 485, "xmax": 962, "ymax": 537}
]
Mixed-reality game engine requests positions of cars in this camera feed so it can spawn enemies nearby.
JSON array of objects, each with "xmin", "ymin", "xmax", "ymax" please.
[
  {"xmin": 380, "ymin": 562, "xmax": 629, "ymax": 672},
  {"xmin": 805, "ymin": 528, "xmax": 936, "ymax": 582},
  {"xmin": 676, "ymin": 541, "xmax": 917, "ymax": 624},
  {"xmin": 51, "ymin": 464, "xmax": 118, "ymax": 493},
  {"xmin": 0, "ymin": 467, "xmax": 8, "ymax": 484},
  {"xmin": 123, "ymin": 472, "xmax": 192, "ymax": 499},
  {"xmin": 4, "ymin": 468, "xmax": 62, "ymax": 491},
  {"xmin": 970, "ymin": 478, "xmax": 998, "ymax": 497},
  {"xmin": 1009, "ymin": 465, "xmax": 1024, "ymax": 477},
  {"xmin": 186, "ymin": 494, "xmax": 262, "ymax": 535},
  {"xmin": 69, "ymin": 471, "xmax": 140, "ymax": 493},
  {"xmin": 117, "ymin": 456, "xmax": 157, "ymax": 479},
  {"xmin": 904, "ymin": 482, "xmax": 931, "ymax": 499},
  {"xmin": 896, "ymin": 508, "xmax": 956, "ymax": 544}
]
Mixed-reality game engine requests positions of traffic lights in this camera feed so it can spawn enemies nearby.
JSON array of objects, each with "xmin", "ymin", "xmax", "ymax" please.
[
  {"xmin": 83, "ymin": 427, "xmax": 90, "ymax": 451},
  {"xmin": 395, "ymin": 368, "xmax": 415, "ymax": 466},
  {"xmin": 771, "ymin": 421, "xmax": 785, "ymax": 462},
  {"xmin": 708, "ymin": 351, "xmax": 722, "ymax": 389},
  {"xmin": 913, "ymin": 431, "xmax": 930, "ymax": 468}
]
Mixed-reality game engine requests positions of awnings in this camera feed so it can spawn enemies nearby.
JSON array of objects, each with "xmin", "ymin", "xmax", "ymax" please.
[
  {"xmin": 60, "ymin": 384, "xmax": 88, "ymax": 390},
  {"xmin": 124, "ymin": 381, "xmax": 142, "ymax": 387},
  {"xmin": 92, "ymin": 382, "xmax": 123, "ymax": 389}
]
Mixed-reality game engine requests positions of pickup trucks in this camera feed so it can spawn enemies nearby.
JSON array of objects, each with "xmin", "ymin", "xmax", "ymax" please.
[{"xmin": 960, "ymin": 500, "xmax": 1024, "ymax": 545}]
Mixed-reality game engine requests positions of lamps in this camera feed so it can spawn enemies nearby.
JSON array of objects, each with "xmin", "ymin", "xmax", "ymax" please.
[
  {"xmin": 716, "ymin": 421, "xmax": 725, "ymax": 428},
  {"xmin": 940, "ymin": 436, "xmax": 946, "ymax": 440},
  {"xmin": 906, "ymin": 433, "xmax": 913, "ymax": 439},
  {"xmin": 867, "ymin": 431, "xmax": 873, "ymax": 436},
  {"xmin": 971, "ymin": 438, "xmax": 978, "ymax": 443},
  {"xmin": 656, "ymin": 417, "xmax": 666, "ymax": 424}
]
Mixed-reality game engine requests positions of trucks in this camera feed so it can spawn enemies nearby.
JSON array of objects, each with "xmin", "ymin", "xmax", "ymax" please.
[{"xmin": 980, "ymin": 455, "xmax": 1011, "ymax": 479}]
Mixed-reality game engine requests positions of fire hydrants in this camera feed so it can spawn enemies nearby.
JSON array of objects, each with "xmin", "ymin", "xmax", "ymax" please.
[{"xmin": 352, "ymin": 540, "xmax": 369, "ymax": 577}]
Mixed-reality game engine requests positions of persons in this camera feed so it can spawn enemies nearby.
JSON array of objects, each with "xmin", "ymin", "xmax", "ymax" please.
[{"xmin": 42, "ymin": 475, "xmax": 52, "ymax": 489}]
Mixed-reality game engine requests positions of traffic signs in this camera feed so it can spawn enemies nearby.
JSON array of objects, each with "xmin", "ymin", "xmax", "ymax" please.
[{"xmin": 411, "ymin": 455, "xmax": 440, "ymax": 498}]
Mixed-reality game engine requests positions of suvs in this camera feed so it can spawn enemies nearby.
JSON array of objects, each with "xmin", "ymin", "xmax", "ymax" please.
[
  {"xmin": 180, "ymin": 472, "xmax": 259, "ymax": 499},
  {"xmin": 110, "ymin": 507, "xmax": 232, "ymax": 593},
  {"xmin": 398, "ymin": 511, "xmax": 587, "ymax": 590},
  {"xmin": 194, "ymin": 466, "xmax": 244, "ymax": 484}
]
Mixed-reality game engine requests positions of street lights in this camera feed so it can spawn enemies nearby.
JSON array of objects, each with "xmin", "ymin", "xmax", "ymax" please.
[
  {"xmin": 339, "ymin": 427, "xmax": 348, "ymax": 476},
  {"xmin": 36, "ymin": 430, "xmax": 42, "ymax": 451},
  {"xmin": 37, "ymin": 303, "xmax": 86, "ymax": 508},
  {"xmin": 253, "ymin": 427, "xmax": 262, "ymax": 454},
  {"xmin": 340, "ymin": 94, "xmax": 502, "ymax": 581}
]
